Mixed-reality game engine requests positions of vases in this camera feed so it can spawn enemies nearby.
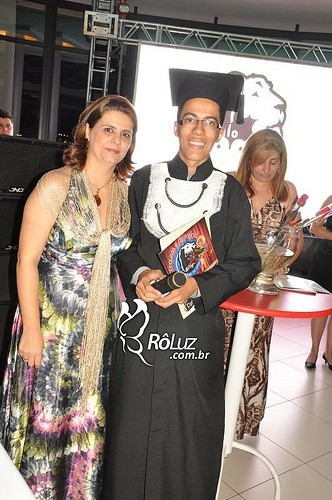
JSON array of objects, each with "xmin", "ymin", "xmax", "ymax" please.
[{"xmin": 245, "ymin": 243, "xmax": 294, "ymax": 296}]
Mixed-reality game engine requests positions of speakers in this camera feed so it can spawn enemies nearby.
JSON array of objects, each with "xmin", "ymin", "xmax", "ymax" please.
[{"xmin": 0, "ymin": 135, "xmax": 72, "ymax": 381}]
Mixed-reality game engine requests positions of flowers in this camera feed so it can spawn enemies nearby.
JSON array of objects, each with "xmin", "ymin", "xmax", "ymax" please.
[{"xmin": 251, "ymin": 194, "xmax": 332, "ymax": 287}]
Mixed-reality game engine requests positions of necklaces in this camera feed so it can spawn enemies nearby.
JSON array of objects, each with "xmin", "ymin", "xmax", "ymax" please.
[{"xmin": 84, "ymin": 169, "xmax": 114, "ymax": 206}]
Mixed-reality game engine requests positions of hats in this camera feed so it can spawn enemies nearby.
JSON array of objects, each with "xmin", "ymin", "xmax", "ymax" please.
[{"xmin": 169, "ymin": 68, "xmax": 245, "ymax": 126}]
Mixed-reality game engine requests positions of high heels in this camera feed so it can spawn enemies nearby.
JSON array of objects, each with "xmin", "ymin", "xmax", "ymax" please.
[
  {"xmin": 323, "ymin": 352, "xmax": 332, "ymax": 371},
  {"xmin": 305, "ymin": 352, "xmax": 318, "ymax": 368}
]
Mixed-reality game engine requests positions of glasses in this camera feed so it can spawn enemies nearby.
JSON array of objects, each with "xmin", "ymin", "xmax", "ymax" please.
[{"xmin": 177, "ymin": 117, "xmax": 222, "ymax": 129}]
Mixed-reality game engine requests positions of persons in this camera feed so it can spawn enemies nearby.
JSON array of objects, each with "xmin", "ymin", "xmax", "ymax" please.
[
  {"xmin": 219, "ymin": 128, "xmax": 304, "ymax": 441},
  {"xmin": 0, "ymin": 95, "xmax": 138, "ymax": 500},
  {"xmin": 290, "ymin": 195, "xmax": 332, "ymax": 370},
  {"xmin": 0, "ymin": 109, "xmax": 14, "ymax": 136},
  {"xmin": 103, "ymin": 69, "xmax": 262, "ymax": 500}
]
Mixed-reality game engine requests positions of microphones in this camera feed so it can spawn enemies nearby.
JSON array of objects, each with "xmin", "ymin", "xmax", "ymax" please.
[{"xmin": 151, "ymin": 272, "xmax": 187, "ymax": 294}]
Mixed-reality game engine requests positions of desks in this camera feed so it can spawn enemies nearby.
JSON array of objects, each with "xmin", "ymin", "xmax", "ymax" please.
[
  {"xmin": 289, "ymin": 235, "xmax": 332, "ymax": 292},
  {"xmin": 213, "ymin": 275, "xmax": 332, "ymax": 500}
]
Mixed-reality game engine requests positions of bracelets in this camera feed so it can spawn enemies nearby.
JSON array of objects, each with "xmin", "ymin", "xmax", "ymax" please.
[{"xmin": 286, "ymin": 264, "xmax": 290, "ymax": 268}]
